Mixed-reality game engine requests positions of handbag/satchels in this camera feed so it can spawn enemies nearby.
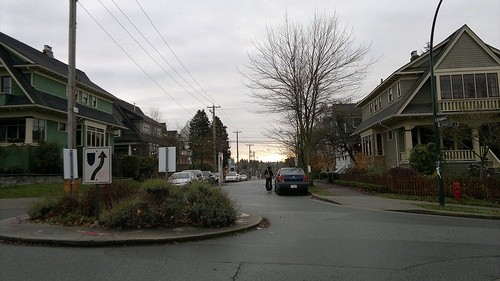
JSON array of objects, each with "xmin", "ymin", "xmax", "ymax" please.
[{"xmin": 265, "ymin": 169, "xmax": 270, "ymax": 179}]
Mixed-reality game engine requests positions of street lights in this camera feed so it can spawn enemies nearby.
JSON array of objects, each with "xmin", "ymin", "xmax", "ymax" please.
[{"xmin": 207, "ymin": 102, "xmax": 221, "ymax": 172}]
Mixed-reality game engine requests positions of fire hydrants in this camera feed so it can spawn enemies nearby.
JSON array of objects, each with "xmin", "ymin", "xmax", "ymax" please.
[{"xmin": 451, "ymin": 182, "xmax": 462, "ymax": 201}]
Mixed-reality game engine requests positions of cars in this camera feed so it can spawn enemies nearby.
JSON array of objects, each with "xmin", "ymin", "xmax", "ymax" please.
[
  {"xmin": 169, "ymin": 171, "xmax": 198, "ymax": 191},
  {"xmin": 214, "ymin": 172, "xmax": 219, "ymax": 184},
  {"xmin": 202, "ymin": 171, "xmax": 214, "ymax": 184},
  {"xmin": 237, "ymin": 174, "xmax": 241, "ymax": 181},
  {"xmin": 241, "ymin": 174, "xmax": 247, "ymax": 181},
  {"xmin": 183, "ymin": 170, "xmax": 204, "ymax": 185},
  {"xmin": 225, "ymin": 172, "xmax": 239, "ymax": 183},
  {"xmin": 275, "ymin": 167, "xmax": 308, "ymax": 195}
]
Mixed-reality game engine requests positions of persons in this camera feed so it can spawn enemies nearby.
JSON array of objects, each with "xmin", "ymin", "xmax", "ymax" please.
[{"xmin": 264, "ymin": 165, "xmax": 274, "ymax": 191}]
